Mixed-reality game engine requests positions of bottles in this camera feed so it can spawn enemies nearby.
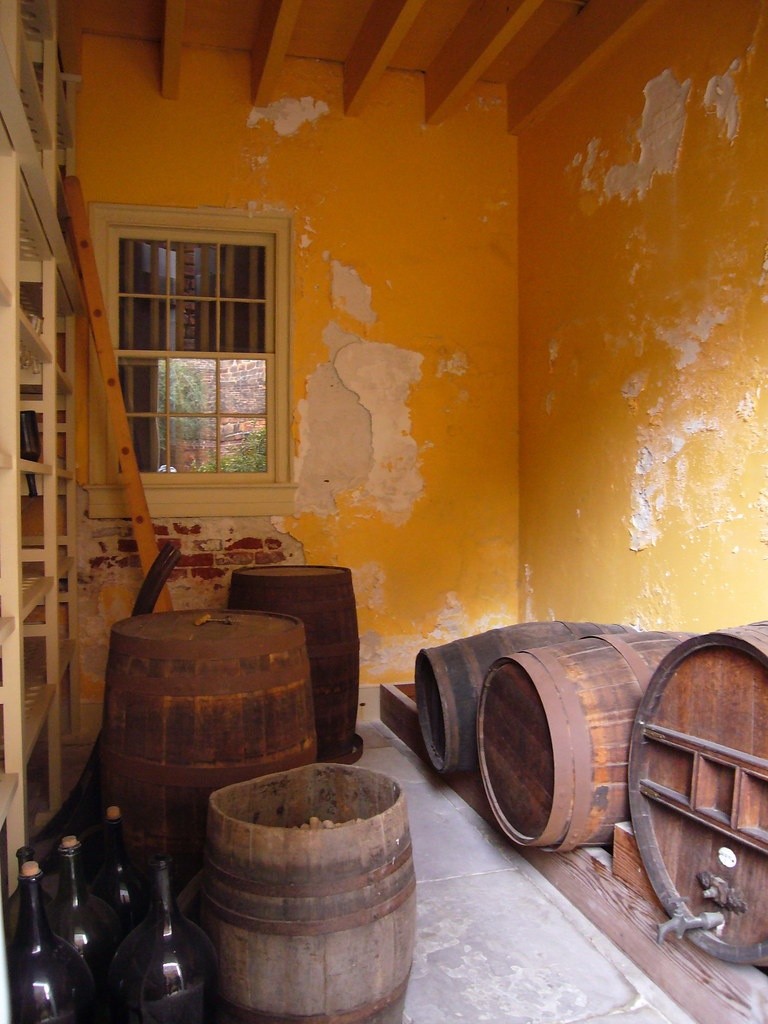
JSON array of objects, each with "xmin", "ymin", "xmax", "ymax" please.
[{"xmin": 3, "ymin": 805, "xmax": 222, "ymax": 1024}]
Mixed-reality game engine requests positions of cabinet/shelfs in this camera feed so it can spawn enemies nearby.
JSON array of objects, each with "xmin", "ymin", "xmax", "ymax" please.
[{"xmin": 0, "ymin": 1, "xmax": 81, "ymax": 943}]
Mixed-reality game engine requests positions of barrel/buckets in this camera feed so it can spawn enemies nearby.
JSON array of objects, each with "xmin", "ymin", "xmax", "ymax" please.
[
  {"xmin": 625, "ymin": 615, "xmax": 768, "ymax": 970},
  {"xmin": 477, "ymin": 626, "xmax": 739, "ymax": 852},
  {"xmin": 413, "ymin": 621, "xmax": 634, "ymax": 779},
  {"xmin": 97, "ymin": 609, "xmax": 323, "ymax": 894},
  {"xmin": 226, "ymin": 560, "xmax": 366, "ymax": 767},
  {"xmin": 207, "ymin": 761, "xmax": 415, "ymax": 1024}
]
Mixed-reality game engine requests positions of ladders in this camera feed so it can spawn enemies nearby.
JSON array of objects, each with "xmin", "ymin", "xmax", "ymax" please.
[{"xmin": 55, "ymin": 169, "xmax": 174, "ymax": 616}]
